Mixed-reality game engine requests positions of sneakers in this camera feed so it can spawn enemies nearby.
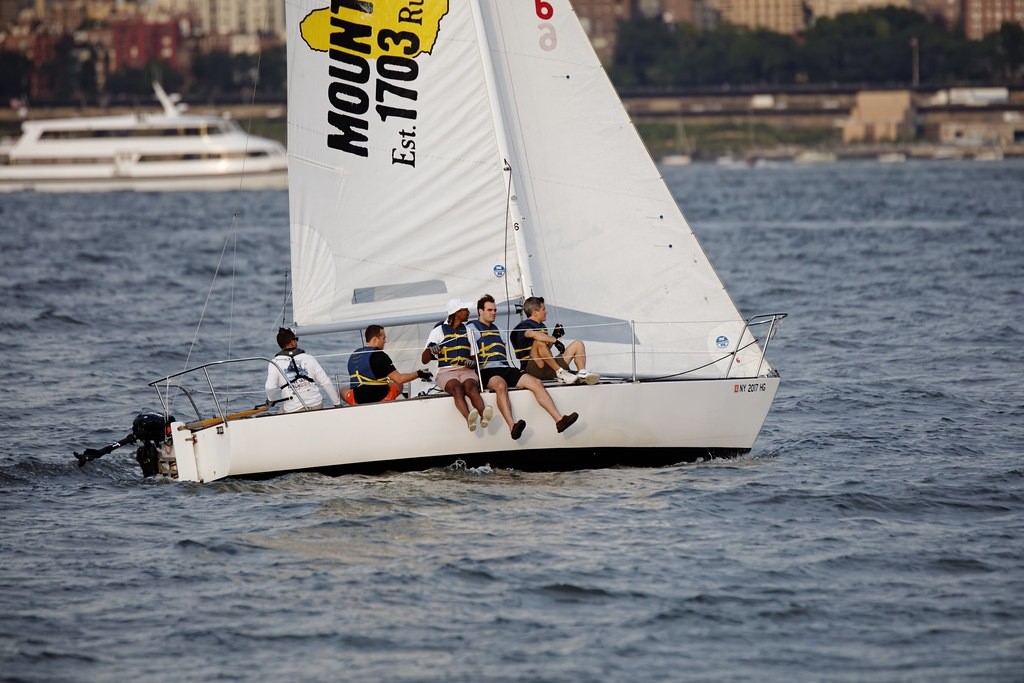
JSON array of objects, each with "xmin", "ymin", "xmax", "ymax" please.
[
  {"xmin": 556, "ymin": 370, "xmax": 578, "ymax": 384},
  {"xmin": 577, "ymin": 370, "xmax": 600, "ymax": 385}
]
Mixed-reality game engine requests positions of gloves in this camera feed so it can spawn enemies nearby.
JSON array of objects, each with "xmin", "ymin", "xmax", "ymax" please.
[
  {"xmin": 427, "ymin": 342, "xmax": 441, "ymax": 355},
  {"xmin": 266, "ymin": 398, "xmax": 276, "ymax": 407},
  {"xmin": 553, "ymin": 340, "xmax": 565, "ymax": 354},
  {"xmin": 464, "ymin": 360, "xmax": 477, "ymax": 369},
  {"xmin": 417, "ymin": 368, "xmax": 433, "ymax": 381},
  {"xmin": 336, "ymin": 402, "xmax": 343, "ymax": 408},
  {"xmin": 552, "ymin": 324, "xmax": 565, "ymax": 339}
]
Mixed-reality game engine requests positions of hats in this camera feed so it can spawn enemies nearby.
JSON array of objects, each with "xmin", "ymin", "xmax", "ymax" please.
[{"xmin": 447, "ymin": 297, "xmax": 474, "ymax": 316}]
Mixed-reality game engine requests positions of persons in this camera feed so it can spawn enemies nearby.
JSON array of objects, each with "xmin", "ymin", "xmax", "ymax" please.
[
  {"xmin": 340, "ymin": 324, "xmax": 433, "ymax": 406},
  {"xmin": 265, "ymin": 327, "xmax": 342, "ymax": 414},
  {"xmin": 510, "ymin": 297, "xmax": 601, "ymax": 384},
  {"xmin": 422, "ymin": 298, "xmax": 492, "ymax": 431},
  {"xmin": 466, "ymin": 293, "xmax": 579, "ymax": 439}
]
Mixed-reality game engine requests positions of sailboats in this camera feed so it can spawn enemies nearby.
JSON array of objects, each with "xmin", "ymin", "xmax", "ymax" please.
[{"xmin": 148, "ymin": 0, "xmax": 790, "ymax": 489}]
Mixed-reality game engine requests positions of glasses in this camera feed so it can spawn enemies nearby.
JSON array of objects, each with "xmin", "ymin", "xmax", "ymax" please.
[{"xmin": 294, "ymin": 337, "xmax": 298, "ymax": 341}]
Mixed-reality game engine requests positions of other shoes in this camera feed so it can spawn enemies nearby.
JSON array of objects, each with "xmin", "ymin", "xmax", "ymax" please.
[
  {"xmin": 556, "ymin": 412, "xmax": 578, "ymax": 433},
  {"xmin": 480, "ymin": 406, "xmax": 493, "ymax": 428},
  {"xmin": 468, "ymin": 409, "xmax": 478, "ymax": 431},
  {"xmin": 511, "ymin": 420, "xmax": 526, "ymax": 440}
]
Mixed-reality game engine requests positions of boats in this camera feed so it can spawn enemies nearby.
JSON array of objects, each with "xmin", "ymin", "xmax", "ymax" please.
[{"xmin": 0, "ymin": 113, "xmax": 288, "ymax": 195}]
